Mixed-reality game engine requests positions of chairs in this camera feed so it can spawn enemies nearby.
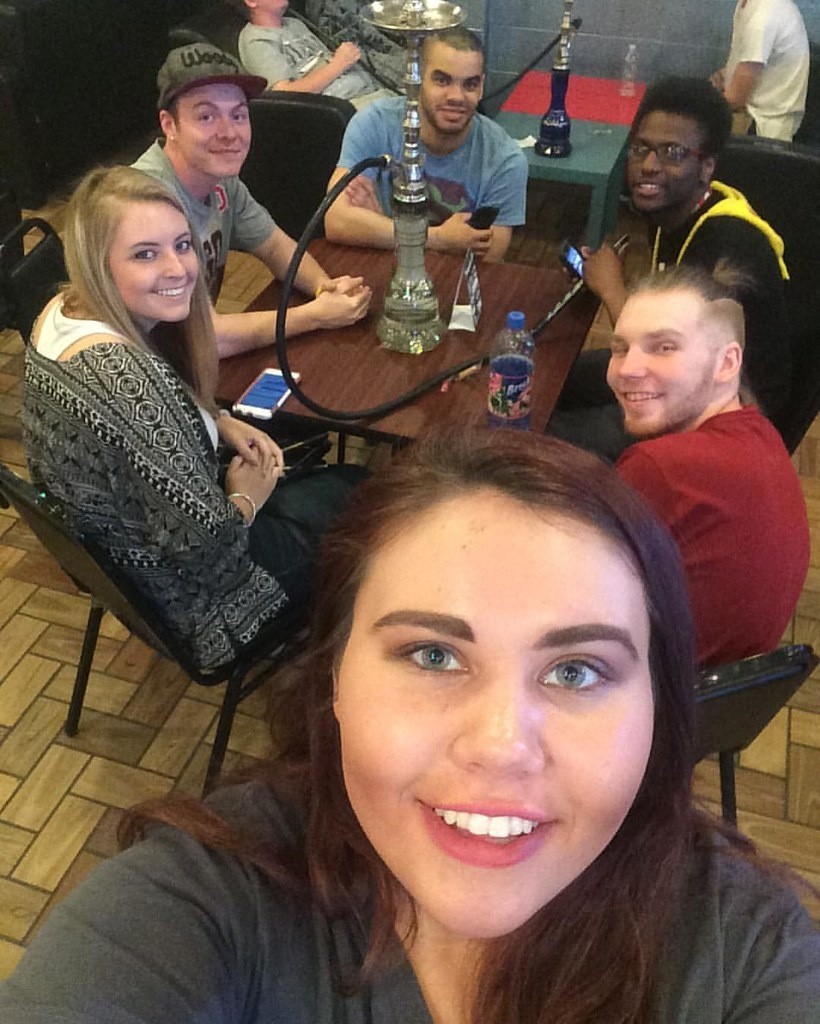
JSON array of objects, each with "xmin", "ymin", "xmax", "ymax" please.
[
  {"xmin": 166, "ymin": 0, "xmax": 357, "ymax": 252},
  {"xmin": 704, "ymin": 40, "xmax": 819, "ymax": 265},
  {"xmin": 0, "ymin": 217, "xmax": 70, "ymax": 345},
  {"xmin": 0, "ymin": 461, "xmax": 317, "ymax": 799},
  {"xmin": 692, "ymin": 641, "xmax": 820, "ymax": 826},
  {"xmin": 770, "ymin": 271, "xmax": 820, "ymax": 457}
]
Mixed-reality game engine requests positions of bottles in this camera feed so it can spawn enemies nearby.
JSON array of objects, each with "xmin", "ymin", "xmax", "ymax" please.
[
  {"xmin": 619, "ymin": 45, "xmax": 638, "ymax": 96},
  {"xmin": 487, "ymin": 311, "xmax": 535, "ymax": 432}
]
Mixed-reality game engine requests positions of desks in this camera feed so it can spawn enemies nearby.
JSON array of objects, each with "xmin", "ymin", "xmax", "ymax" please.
[
  {"xmin": 213, "ymin": 238, "xmax": 602, "ymax": 477},
  {"xmin": 492, "ymin": 68, "xmax": 655, "ymax": 250}
]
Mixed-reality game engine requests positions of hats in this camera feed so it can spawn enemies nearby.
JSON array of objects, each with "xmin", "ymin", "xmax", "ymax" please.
[{"xmin": 156, "ymin": 43, "xmax": 268, "ymax": 109}]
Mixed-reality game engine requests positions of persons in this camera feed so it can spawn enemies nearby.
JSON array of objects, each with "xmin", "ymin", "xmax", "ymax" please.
[
  {"xmin": 238, "ymin": 0, "xmax": 400, "ymax": 112},
  {"xmin": 23, "ymin": 164, "xmax": 384, "ymax": 676},
  {"xmin": 0, "ymin": 404, "xmax": 819, "ymax": 1023},
  {"xmin": 324, "ymin": 24, "xmax": 528, "ymax": 263},
  {"xmin": 607, "ymin": 258, "xmax": 811, "ymax": 671},
  {"xmin": 562, "ymin": 73, "xmax": 820, "ymax": 456},
  {"xmin": 128, "ymin": 40, "xmax": 372, "ymax": 359},
  {"xmin": 708, "ymin": 1, "xmax": 808, "ymax": 141}
]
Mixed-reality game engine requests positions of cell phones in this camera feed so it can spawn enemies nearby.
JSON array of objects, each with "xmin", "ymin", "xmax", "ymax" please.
[
  {"xmin": 467, "ymin": 206, "xmax": 500, "ymax": 231},
  {"xmin": 233, "ymin": 367, "xmax": 303, "ymax": 420},
  {"xmin": 560, "ymin": 239, "xmax": 584, "ymax": 281}
]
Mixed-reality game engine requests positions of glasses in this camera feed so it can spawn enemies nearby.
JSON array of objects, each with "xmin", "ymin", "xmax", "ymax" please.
[{"xmin": 627, "ymin": 140, "xmax": 708, "ymax": 164}]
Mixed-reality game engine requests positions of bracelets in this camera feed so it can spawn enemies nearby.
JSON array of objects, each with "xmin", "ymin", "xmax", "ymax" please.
[
  {"xmin": 315, "ymin": 284, "xmax": 322, "ymax": 298},
  {"xmin": 227, "ymin": 492, "xmax": 256, "ymax": 528}
]
